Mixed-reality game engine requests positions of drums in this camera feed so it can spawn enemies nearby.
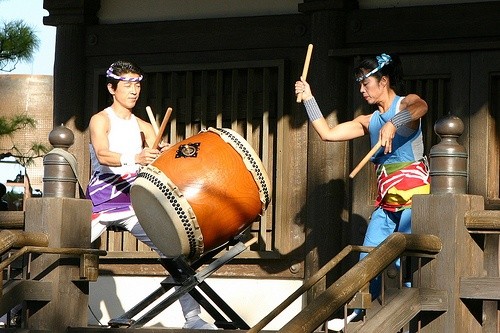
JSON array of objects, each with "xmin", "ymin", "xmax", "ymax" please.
[{"xmin": 129, "ymin": 127, "xmax": 271, "ymax": 257}]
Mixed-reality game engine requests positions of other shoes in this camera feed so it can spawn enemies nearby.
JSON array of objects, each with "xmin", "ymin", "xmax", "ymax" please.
[
  {"xmin": 182, "ymin": 321, "xmax": 218, "ymax": 330},
  {"xmin": 326, "ymin": 307, "xmax": 360, "ymax": 331}
]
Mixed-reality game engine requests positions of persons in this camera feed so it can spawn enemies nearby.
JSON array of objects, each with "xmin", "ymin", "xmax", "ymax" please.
[
  {"xmin": 85, "ymin": 62, "xmax": 216, "ymax": 330},
  {"xmin": 295, "ymin": 53, "xmax": 431, "ymax": 331}
]
